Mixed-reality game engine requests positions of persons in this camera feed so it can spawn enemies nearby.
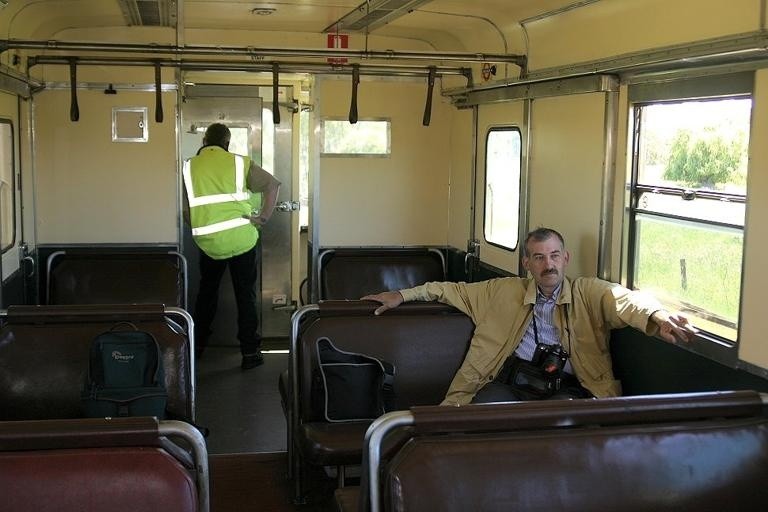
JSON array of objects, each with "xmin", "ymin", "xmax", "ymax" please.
[
  {"xmin": 360, "ymin": 226, "xmax": 698, "ymax": 405},
  {"xmin": 184, "ymin": 122, "xmax": 283, "ymax": 369}
]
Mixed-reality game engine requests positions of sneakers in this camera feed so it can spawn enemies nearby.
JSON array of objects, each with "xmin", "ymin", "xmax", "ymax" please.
[{"xmin": 239, "ymin": 351, "xmax": 265, "ymax": 371}]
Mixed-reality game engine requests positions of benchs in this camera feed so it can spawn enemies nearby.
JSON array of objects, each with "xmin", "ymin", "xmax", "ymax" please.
[
  {"xmin": 315, "ymin": 248, "xmax": 447, "ymax": 302},
  {"xmin": 280, "ymin": 299, "xmax": 476, "ymax": 512},
  {"xmin": 334, "ymin": 391, "xmax": 767, "ymax": 512},
  {"xmin": 0, "ymin": 417, "xmax": 213, "ymax": 510},
  {"xmin": 17, "ymin": 243, "xmax": 189, "ymax": 333},
  {"xmin": 0, "ymin": 304, "xmax": 195, "ymax": 426}
]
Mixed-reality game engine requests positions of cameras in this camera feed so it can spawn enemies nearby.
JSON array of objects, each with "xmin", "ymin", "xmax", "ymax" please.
[{"xmin": 532, "ymin": 343, "xmax": 568, "ymax": 378}]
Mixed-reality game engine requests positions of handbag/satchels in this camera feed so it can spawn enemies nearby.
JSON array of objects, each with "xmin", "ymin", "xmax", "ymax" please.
[{"xmin": 315, "ymin": 336, "xmax": 396, "ymax": 423}]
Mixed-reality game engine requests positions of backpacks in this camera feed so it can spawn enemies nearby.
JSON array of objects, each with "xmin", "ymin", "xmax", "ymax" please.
[{"xmin": 79, "ymin": 319, "xmax": 210, "ymax": 442}]
